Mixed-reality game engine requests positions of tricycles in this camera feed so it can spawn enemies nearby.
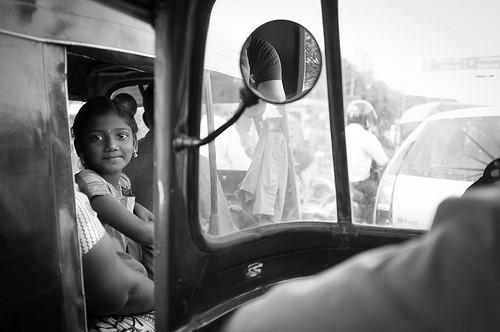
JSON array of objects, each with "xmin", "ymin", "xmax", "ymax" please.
[{"xmin": 0, "ymin": 0, "xmax": 299, "ymax": 332}]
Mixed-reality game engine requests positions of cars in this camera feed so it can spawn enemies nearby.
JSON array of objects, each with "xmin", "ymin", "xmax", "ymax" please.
[{"xmin": 372, "ymin": 102, "xmax": 500, "ymax": 230}]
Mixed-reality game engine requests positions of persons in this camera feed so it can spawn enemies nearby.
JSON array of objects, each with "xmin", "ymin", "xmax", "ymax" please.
[
  {"xmin": 241, "ymin": 35, "xmax": 286, "ymax": 104},
  {"xmin": 199, "ymin": 99, "xmax": 390, "ymax": 205},
  {"xmin": 70, "ymin": 81, "xmax": 154, "ymax": 332},
  {"xmin": 220, "ymin": 182, "xmax": 500, "ymax": 332}
]
